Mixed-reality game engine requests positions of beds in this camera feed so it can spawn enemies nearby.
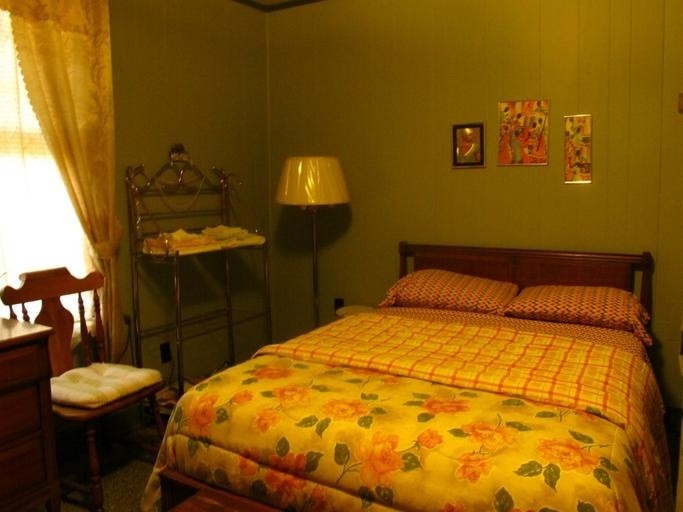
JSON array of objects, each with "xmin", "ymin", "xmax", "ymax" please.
[{"xmin": 158, "ymin": 242, "xmax": 659, "ymax": 512}]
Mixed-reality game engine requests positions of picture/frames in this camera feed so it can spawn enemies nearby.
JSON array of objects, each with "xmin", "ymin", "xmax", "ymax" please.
[
  {"xmin": 449, "ymin": 121, "xmax": 486, "ymax": 170},
  {"xmin": 496, "ymin": 97, "xmax": 549, "ymax": 166},
  {"xmin": 561, "ymin": 113, "xmax": 593, "ymax": 185}
]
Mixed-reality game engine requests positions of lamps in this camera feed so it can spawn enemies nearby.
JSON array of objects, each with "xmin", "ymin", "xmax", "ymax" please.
[{"xmin": 274, "ymin": 156, "xmax": 347, "ymax": 332}]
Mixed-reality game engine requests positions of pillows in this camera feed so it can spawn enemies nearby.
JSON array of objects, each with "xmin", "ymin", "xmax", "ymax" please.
[{"xmin": 377, "ymin": 269, "xmax": 652, "ymax": 347}]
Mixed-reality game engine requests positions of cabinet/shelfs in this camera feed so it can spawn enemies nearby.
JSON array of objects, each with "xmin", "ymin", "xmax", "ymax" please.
[{"xmin": 126, "ymin": 141, "xmax": 272, "ymax": 467}]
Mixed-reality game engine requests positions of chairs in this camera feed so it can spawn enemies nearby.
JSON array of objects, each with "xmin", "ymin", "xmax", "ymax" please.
[{"xmin": 0, "ymin": 266, "xmax": 166, "ymax": 511}]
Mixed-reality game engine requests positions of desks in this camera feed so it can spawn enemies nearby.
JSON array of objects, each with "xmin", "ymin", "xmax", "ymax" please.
[{"xmin": 0, "ymin": 316, "xmax": 60, "ymax": 512}]
